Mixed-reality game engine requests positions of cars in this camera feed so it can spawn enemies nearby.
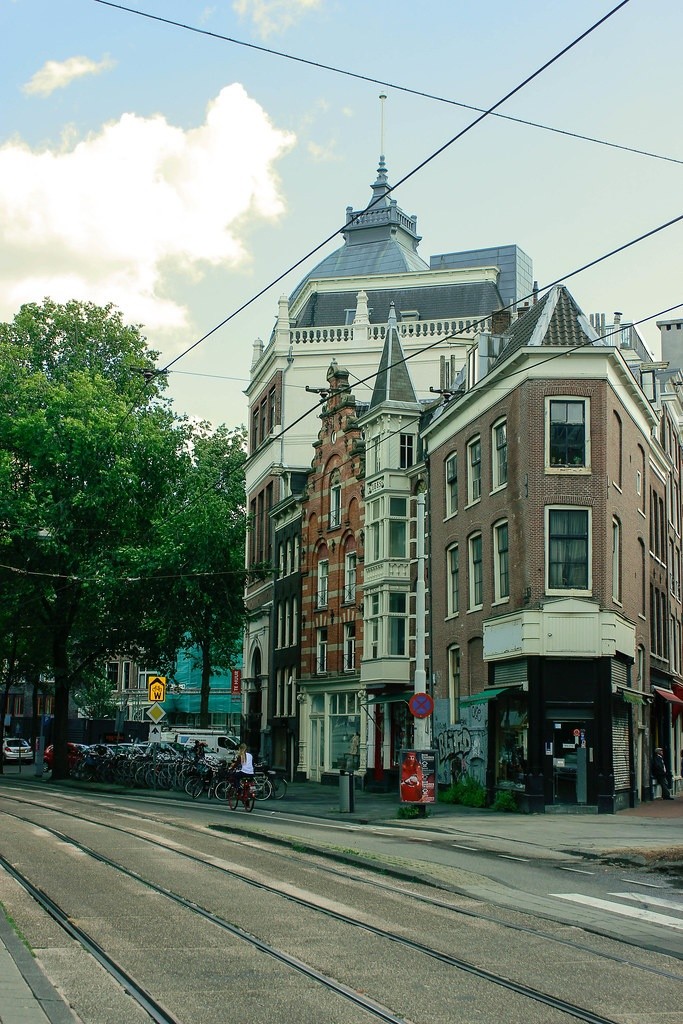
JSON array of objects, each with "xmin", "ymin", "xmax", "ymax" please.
[
  {"xmin": 1, "ymin": 737, "xmax": 35, "ymax": 766},
  {"xmin": 41, "ymin": 742, "xmax": 227, "ymax": 774}
]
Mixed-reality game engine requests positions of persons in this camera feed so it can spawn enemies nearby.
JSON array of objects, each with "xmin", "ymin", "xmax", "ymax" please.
[
  {"xmin": 230, "ymin": 743, "xmax": 255, "ymax": 796},
  {"xmin": 191, "ymin": 740, "xmax": 205, "ymax": 762},
  {"xmin": 401, "ymin": 753, "xmax": 422, "ymax": 800},
  {"xmin": 652, "ymin": 748, "xmax": 674, "ymax": 800}
]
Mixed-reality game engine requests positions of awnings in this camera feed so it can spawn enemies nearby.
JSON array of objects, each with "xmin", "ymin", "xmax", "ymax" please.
[
  {"xmin": 359, "ymin": 693, "xmax": 414, "ymax": 706},
  {"xmin": 457, "ymin": 687, "xmax": 509, "ymax": 709},
  {"xmin": 651, "ymin": 685, "xmax": 683, "ymax": 703}
]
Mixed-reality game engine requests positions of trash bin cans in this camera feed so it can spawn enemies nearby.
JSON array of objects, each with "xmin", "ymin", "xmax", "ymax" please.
[{"xmin": 339, "ymin": 769, "xmax": 356, "ymax": 813}]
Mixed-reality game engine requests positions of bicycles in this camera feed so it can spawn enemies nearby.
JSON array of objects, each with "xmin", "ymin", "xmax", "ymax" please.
[{"xmin": 69, "ymin": 742, "xmax": 288, "ymax": 813}]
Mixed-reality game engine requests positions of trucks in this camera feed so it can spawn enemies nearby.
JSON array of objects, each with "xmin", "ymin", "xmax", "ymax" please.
[{"xmin": 175, "ymin": 729, "xmax": 242, "ymax": 763}]
